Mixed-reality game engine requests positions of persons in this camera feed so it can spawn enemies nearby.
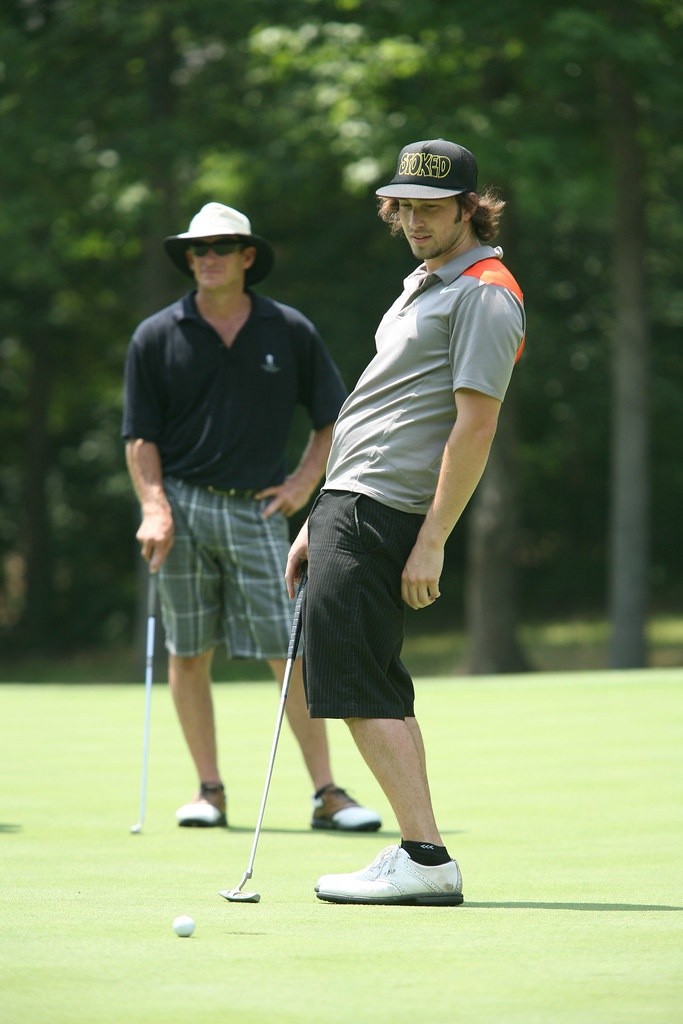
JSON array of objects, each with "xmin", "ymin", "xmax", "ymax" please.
[
  {"xmin": 117, "ymin": 198, "xmax": 382, "ymax": 833},
  {"xmin": 281, "ymin": 137, "xmax": 525, "ymax": 906}
]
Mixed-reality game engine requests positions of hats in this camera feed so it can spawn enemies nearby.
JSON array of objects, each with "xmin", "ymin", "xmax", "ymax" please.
[
  {"xmin": 375, "ymin": 139, "xmax": 477, "ymax": 199},
  {"xmin": 161, "ymin": 202, "xmax": 273, "ymax": 286}
]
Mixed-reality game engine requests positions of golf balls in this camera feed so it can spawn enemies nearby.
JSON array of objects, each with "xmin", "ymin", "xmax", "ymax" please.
[{"xmin": 173, "ymin": 917, "xmax": 195, "ymax": 938}]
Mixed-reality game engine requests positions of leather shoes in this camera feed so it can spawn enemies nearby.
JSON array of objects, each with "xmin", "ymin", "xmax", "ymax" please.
[
  {"xmin": 177, "ymin": 798, "xmax": 227, "ymax": 827},
  {"xmin": 311, "ymin": 789, "xmax": 381, "ymax": 830},
  {"xmin": 314, "ymin": 845, "xmax": 463, "ymax": 906}
]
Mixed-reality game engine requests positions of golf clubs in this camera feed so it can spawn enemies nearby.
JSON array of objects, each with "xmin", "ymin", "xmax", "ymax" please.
[
  {"xmin": 222, "ymin": 566, "xmax": 307, "ymax": 907},
  {"xmin": 126, "ymin": 559, "xmax": 157, "ymax": 834}
]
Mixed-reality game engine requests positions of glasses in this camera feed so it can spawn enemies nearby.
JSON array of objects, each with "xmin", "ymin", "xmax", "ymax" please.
[{"xmin": 190, "ymin": 238, "xmax": 248, "ymax": 257}]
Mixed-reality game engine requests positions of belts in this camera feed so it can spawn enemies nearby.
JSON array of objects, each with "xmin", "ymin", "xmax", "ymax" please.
[{"xmin": 208, "ymin": 483, "xmax": 254, "ymax": 502}]
